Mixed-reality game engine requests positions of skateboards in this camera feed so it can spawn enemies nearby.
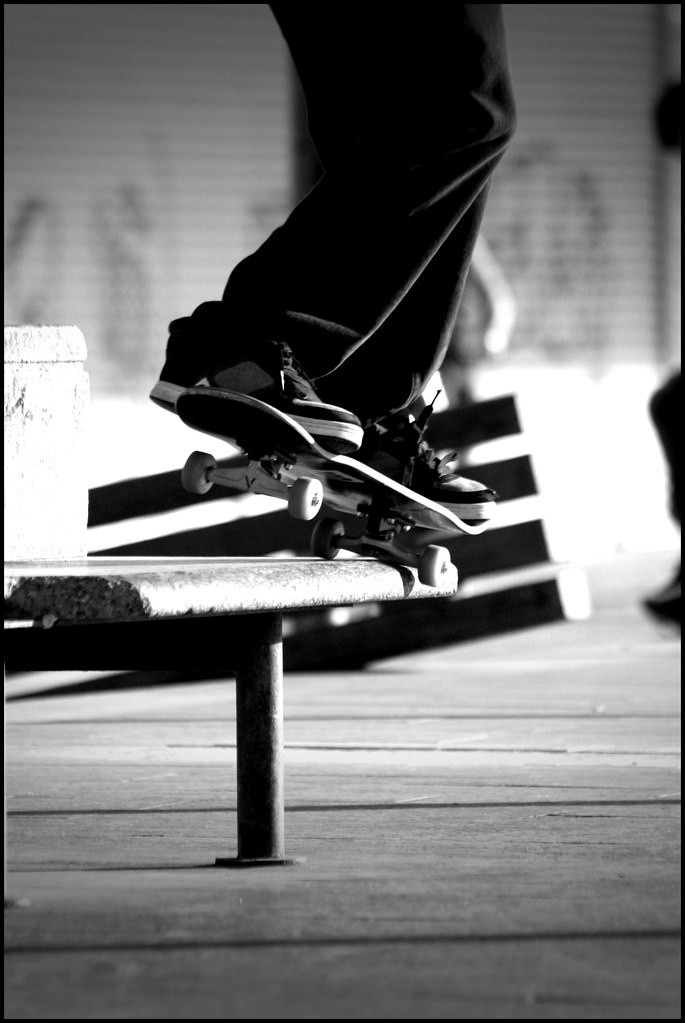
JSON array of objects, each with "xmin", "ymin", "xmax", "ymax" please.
[{"xmin": 176, "ymin": 387, "xmax": 491, "ymax": 588}]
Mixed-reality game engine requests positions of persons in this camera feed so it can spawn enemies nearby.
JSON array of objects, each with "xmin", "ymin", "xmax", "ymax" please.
[
  {"xmin": 439, "ymin": 233, "xmax": 516, "ymax": 410},
  {"xmin": 643, "ymin": 79, "xmax": 685, "ymax": 631},
  {"xmin": 148, "ymin": 0, "xmax": 518, "ymax": 526}
]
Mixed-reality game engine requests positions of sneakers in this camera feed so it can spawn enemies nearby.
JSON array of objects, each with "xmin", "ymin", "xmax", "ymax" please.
[
  {"xmin": 344, "ymin": 389, "xmax": 498, "ymax": 522},
  {"xmin": 149, "ymin": 314, "xmax": 364, "ymax": 455}
]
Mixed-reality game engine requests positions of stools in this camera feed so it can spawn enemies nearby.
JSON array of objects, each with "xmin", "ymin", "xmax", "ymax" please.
[{"xmin": 3, "ymin": 560, "xmax": 462, "ymax": 871}]
